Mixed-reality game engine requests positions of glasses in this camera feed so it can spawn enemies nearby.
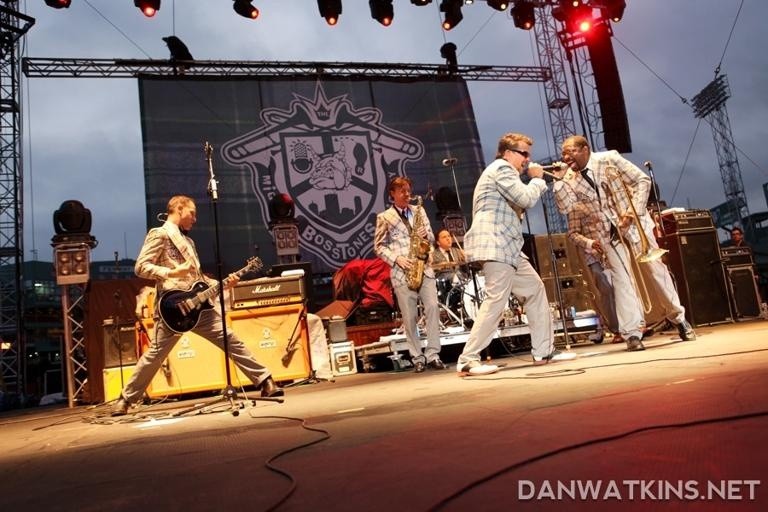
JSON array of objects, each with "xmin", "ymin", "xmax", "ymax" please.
[
  {"xmin": 732, "ymin": 233, "xmax": 740, "ymax": 236},
  {"xmin": 511, "ymin": 148, "xmax": 530, "ymax": 159}
]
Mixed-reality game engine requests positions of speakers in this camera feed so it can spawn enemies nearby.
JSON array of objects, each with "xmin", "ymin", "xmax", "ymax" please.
[
  {"xmin": 103, "ymin": 326, "xmax": 136, "ymax": 368},
  {"xmin": 657, "ymin": 230, "xmax": 739, "ymax": 325},
  {"xmin": 726, "ymin": 266, "xmax": 765, "ymax": 321},
  {"xmin": 584, "ymin": 23, "xmax": 632, "ymax": 153},
  {"xmin": 535, "ymin": 275, "xmax": 588, "ymax": 311},
  {"xmin": 522, "ymin": 230, "xmax": 584, "ymax": 277}
]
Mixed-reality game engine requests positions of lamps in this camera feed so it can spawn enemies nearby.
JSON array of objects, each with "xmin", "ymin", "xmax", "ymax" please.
[{"xmin": 41, "ymin": 0, "xmax": 561, "ymax": 32}]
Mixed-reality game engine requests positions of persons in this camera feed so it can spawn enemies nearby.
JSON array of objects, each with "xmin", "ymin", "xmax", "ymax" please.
[
  {"xmin": 110, "ymin": 195, "xmax": 284, "ymax": 417},
  {"xmin": 552, "ymin": 135, "xmax": 696, "ymax": 352},
  {"xmin": 431, "ymin": 228, "xmax": 465, "ymax": 324},
  {"xmin": 373, "ymin": 176, "xmax": 449, "ymax": 373},
  {"xmin": 456, "ymin": 132, "xmax": 578, "ymax": 377},
  {"xmin": 728, "ymin": 228, "xmax": 755, "ymax": 264},
  {"xmin": 567, "ymin": 201, "xmax": 654, "ymax": 343}
]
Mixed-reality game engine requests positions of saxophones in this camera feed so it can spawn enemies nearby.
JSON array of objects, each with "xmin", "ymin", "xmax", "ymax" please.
[{"xmin": 404, "ymin": 197, "xmax": 429, "ymax": 289}]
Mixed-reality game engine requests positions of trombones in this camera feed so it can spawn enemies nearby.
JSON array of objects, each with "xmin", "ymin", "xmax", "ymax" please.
[{"xmin": 604, "ymin": 163, "xmax": 653, "ymax": 314}]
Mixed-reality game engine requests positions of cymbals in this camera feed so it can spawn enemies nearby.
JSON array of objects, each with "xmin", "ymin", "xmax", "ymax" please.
[{"xmin": 429, "ymin": 261, "xmax": 460, "ymax": 268}]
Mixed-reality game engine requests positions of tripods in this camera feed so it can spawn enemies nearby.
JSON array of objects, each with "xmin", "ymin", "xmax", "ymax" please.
[
  {"xmin": 87, "ymin": 300, "xmax": 177, "ymax": 410},
  {"xmin": 170, "ymin": 200, "xmax": 283, "ymax": 417},
  {"xmin": 283, "ymin": 299, "xmax": 336, "ymax": 388}
]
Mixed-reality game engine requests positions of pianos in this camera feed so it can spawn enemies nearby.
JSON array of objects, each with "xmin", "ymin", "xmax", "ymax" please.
[{"xmin": 720, "ymin": 247, "xmax": 751, "ymax": 256}]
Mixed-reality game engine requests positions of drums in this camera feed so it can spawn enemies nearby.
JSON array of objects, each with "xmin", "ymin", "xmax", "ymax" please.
[{"xmin": 446, "ymin": 274, "xmax": 486, "ymax": 326}]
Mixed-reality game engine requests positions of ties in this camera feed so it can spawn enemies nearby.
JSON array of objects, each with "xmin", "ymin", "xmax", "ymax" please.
[
  {"xmin": 445, "ymin": 250, "xmax": 453, "ymax": 262},
  {"xmin": 401, "ymin": 210, "xmax": 412, "ymax": 233},
  {"xmin": 580, "ymin": 168, "xmax": 600, "ymax": 196}
]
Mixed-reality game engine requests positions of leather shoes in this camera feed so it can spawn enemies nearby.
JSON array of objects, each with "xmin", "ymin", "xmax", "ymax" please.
[
  {"xmin": 532, "ymin": 350, "xmax": 576, "ymax": 361},
  {"xmin": 430, "ymin": 360, "xmax": 449, "ymax": 370},
  {"xmin": 626, "ymin": 335, "xmax": 645, "ymax": 352},
  {"xmin": 110, "ymin": 393, "xmax": 129, "ymax": 417},
  {"xmin": 676, "ymin": 319, "xmax": 697, "ymax": 341},
  {"xmin": 260, "ymin": 377, "xmax": 284, "ymax": 397},
  {"xmin": 414, "ymin": 362, "xmax": 425, "ymax": 373},
  {"xmin": 456, "ymin": 361, "xmax": 500, "ymax": 376}
]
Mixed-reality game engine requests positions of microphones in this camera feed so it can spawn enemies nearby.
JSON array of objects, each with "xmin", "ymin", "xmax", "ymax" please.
[
  {"xmin": 165, "ymin": 360, "xmax": 173, "ymax": 385},
  {"xmin": 282, "ymin": 348, "xmax": 298, "ymax": 368},
  {"xmin": 543, "ymin": 163, "xmax": 568, "ymax": 170},
  {"xmin": 527, "ymin": 162, "xmax": 562, "ymax": 181},
  {"xmin": 442, "ymin": 158, "xmax": 457, "ymax": 166}
]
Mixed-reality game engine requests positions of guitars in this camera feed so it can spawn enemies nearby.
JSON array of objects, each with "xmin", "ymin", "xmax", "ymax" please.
[{"xmin": 159, "ymin": 255, "xmax": 262, "ymax": 333}]
